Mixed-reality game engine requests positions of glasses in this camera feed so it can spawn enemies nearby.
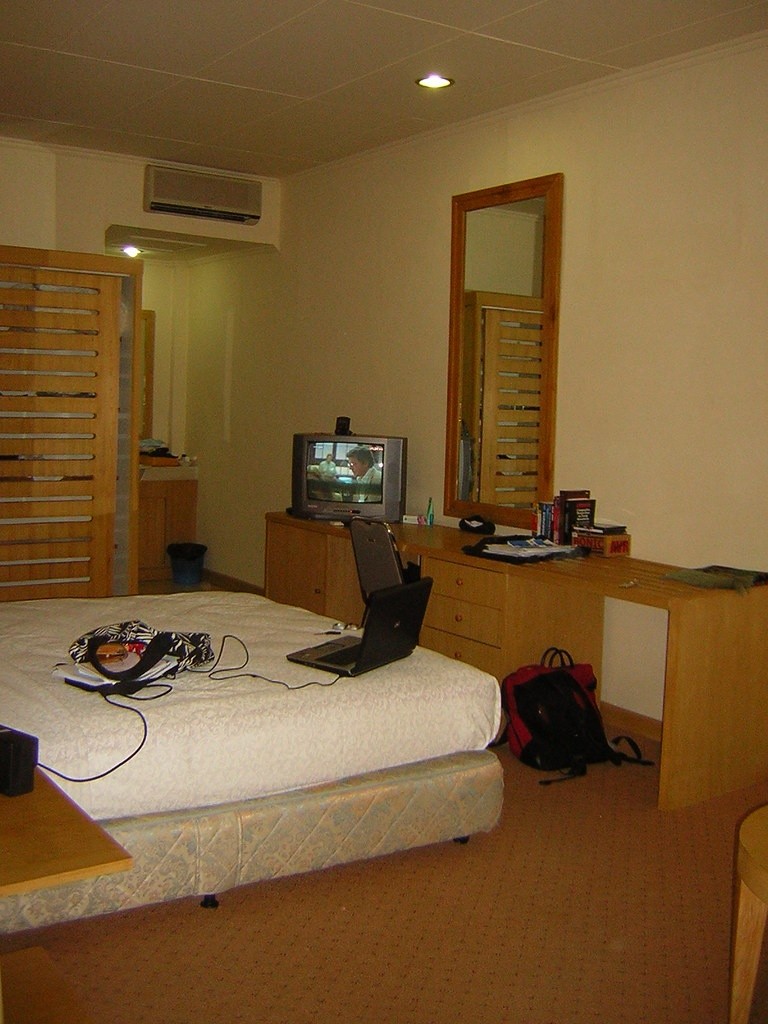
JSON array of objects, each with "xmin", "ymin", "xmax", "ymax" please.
[{"xmin": 347, "ymin": 462, "xmax": 356, "ymax": 467}]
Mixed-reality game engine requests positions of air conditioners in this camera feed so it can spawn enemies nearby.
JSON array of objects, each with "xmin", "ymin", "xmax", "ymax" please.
[{"xmin": 143, "ymin": 164, "xmax": 262, "ymax": 226}]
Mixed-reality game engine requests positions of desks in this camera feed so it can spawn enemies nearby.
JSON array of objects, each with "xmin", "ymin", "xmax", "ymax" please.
[{"xmin": 0, "ymin": 767, "xmax": 137, "ymax": 899}]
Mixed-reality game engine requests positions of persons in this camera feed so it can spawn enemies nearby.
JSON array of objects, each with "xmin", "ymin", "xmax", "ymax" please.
[{"xmin": 307, "ymin": 442, "xmax": 383, "ymax": 502}]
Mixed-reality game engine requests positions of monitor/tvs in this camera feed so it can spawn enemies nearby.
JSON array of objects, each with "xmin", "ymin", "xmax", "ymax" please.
[{"xmin": 291, "ymin": 431, "xmax": 409, "ymax": 523}]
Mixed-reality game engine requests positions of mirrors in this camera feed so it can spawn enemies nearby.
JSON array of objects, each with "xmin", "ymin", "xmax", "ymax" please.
[{"xmin": 441, "ymin": 173, "xmax": 565, "ymax": 531}]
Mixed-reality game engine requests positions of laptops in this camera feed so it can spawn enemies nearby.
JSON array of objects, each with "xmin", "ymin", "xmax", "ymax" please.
[{"xmin": 286, "ymin": 576, "xmax": 433, "ymax": 677}]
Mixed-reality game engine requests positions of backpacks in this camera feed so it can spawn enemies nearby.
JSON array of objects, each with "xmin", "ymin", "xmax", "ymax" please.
[{"xmin": 489, "ymin": 672, "xmax": 656, "ymax": 785}]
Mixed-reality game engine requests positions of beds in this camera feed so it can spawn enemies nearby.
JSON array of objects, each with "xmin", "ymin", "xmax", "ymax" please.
[{"xmin": 0, "ymin": 590, "xmax": 506, "ymax": 937}]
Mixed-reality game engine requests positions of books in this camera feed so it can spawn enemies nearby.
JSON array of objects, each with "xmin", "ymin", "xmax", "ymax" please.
[
  {"xmin": 49, "ymin": 641, "xmax": 180, "ymax": 688},
  {"xmin": 481, "ymin": 488, "xmax": 627, "ymax": 560}
]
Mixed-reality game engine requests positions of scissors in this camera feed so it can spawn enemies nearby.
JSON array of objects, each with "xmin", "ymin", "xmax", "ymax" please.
[{"xmin": 314, "ymin": 631, "xmax": 341, "ymax": 635}]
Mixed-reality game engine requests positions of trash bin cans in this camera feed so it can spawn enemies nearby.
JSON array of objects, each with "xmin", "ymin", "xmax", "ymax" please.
[{"xmin": 167, "ymin": 542, "xmax": 208, "ymax": 585}]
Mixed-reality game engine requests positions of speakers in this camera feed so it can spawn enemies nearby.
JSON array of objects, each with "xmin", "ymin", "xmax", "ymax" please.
[{"xmin": 334, "ymin": 417, "xmax": 351, "ymax": 435}]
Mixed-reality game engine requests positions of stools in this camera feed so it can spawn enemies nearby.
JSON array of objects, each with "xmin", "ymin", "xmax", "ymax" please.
[{"xmin": 728, "ymin": 804, "xmax": 768, "ymax": 1024}]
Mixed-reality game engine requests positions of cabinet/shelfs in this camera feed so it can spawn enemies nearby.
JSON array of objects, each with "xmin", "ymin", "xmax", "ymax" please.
[
  {"xmin": 264, "ymin": 510, "xmax": 455, "ymax": 629},
  {"xmin": 419, "ymin": 530, "xmax": 606, "ymax": 710},
  {"xmin": 461, "ymin": 290, "xmax": 544, "ymax": 513},
  {"xmin": 138, "ymin": 481, "xmax": 198, "ymax": 581},
  {"xmin": 0, "ymin": 244, "xmax": 144, "ymax": 603}
]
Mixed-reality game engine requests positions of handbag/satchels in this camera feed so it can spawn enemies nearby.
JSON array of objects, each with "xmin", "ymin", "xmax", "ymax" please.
[
  {"xmin": 517, "ymin": 647, "xmax": 604, "ymax": 718},
  {"xmin": 69, "ymin": 620, "xmax": 215, "ymax": 696}
]
